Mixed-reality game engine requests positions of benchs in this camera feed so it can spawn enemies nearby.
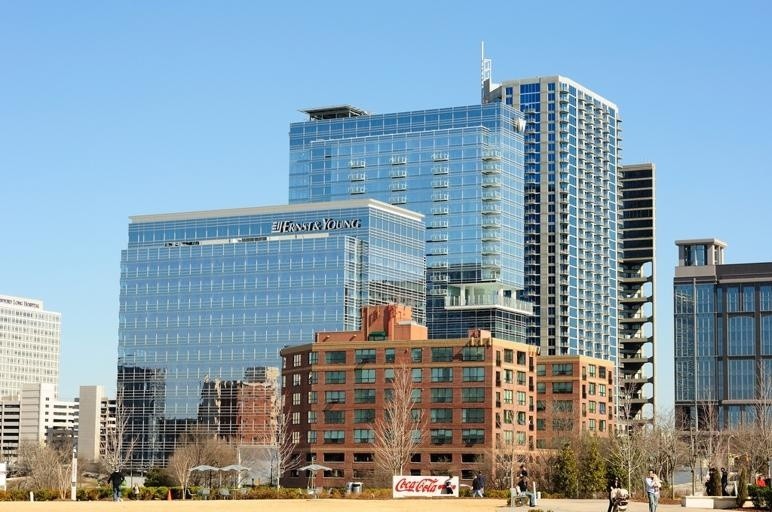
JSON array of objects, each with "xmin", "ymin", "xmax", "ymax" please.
[{"xmin": 509, "ymin": 487, "xmax": 528, "ymax": 508}]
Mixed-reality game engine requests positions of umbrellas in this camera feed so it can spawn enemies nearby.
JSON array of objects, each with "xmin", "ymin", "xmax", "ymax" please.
[
  {"xmin": 186, "ymin": 464, "xmax": 220, "ymax": 485},
  {"xmin": 220, "ymin": 464, "xmax": 252, "ymax": 489},
  {"xmin": 296, "ymin": 463, "xmax": 333, "ymax": 489}
]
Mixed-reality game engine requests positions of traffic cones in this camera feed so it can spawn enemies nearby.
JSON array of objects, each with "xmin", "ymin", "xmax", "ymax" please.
[{"xmin": 166, "ymin": 490, "xmax": 172, "ymax": 500}]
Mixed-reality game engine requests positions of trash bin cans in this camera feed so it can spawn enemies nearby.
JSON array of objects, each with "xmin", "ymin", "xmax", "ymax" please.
[
  {"xmin": 536, "ymin": 491, "xmax": 542, "ymax": 499},
  {"xmin": 345, "ymin": 481, "xmax": 363, "ymax": 494}
]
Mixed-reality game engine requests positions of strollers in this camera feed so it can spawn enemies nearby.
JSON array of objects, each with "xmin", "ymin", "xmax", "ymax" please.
[{"xmin": 611, "ymin": 488, "xmax": 630, "ymax": 512}]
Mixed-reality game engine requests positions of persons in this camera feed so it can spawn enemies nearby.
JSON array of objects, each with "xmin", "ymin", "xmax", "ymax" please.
[
  {"xmin": 107, "ymin": 467, "xmax": 125, "ymax": 502},
  {"xmin": 133, "ymin": 484, "xmax": 140, "ymax": 500},
  {"xmin": 705, "ymin": 467, "xmax": 730, "ymax": 496},
  {"xmin": 477, "ymin": 471, "xmax": 485, "ymax": 494},
  {"xmin": 518, "ymin": 471, "xmax": 536, "ymax": 507},
  {"xmin": 755, "ymin": 476, "xmax": 765, "ymax": 486},
  {"xmin": 252, "ymin": 478, "xmax": 256, "ymax": 487},
  {"xmin": 471, "ymin": 475, "xmax": 484, "ymax": 498},
  {"xmin": 444, "ymin": 474, "xmax": 454, "ymax": 494},
  {"xmin": 644, "ymin": 469, "xmax": 660, "ymax": 512},
  {"xmin": 607, "ymin": 476, "xmax": 622, "ymax": 512},
  {"xmin": 519, "ymin": 465, "xmax": 527, "ymax": 477}
]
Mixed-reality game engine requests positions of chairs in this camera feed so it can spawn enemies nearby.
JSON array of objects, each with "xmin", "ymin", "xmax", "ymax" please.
[{"xmin": 187, "ymin": 485, "xmax": 233, "ymax": 500}]
